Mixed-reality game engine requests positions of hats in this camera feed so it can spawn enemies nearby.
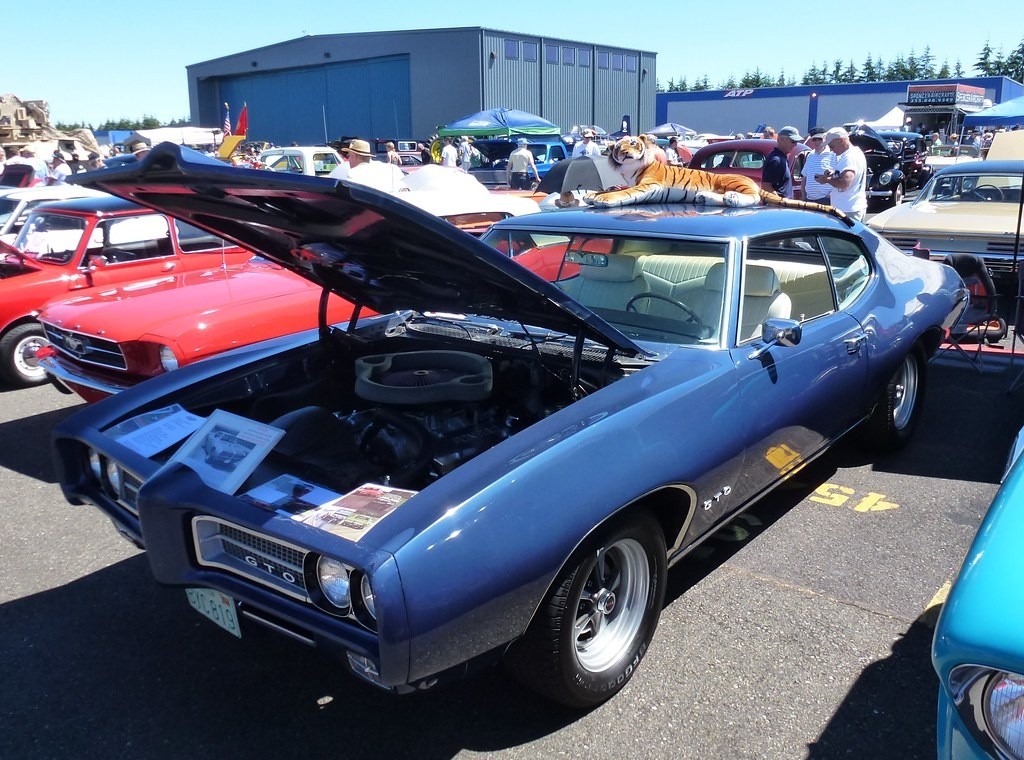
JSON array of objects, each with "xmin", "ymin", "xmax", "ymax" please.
[
  {"xmin": 516, "ymin": 138, "xmax": 529, "ymax": 144},
  {"xmin": 341, "ymin": 139, "xmax": 378, "ymax": 158},
  {"xmin": 820, "ymin": 126, "xmax": 849, "ymax": 146},
  {"xmin": 461, "ymin": 135, "xmax": 468, "ymax": 141},
  {"xmin": 190, "ymin": 143, "xmax": 201, "ymax": 150},
  {"xmin": 951, "ymin": 136, "xmax": 957, "ymax": 137},
  {"xmin": 291, "ymin": 141, "xmax": 297, "ymax": 146},
  {"xmin": 52, "ymin": 153, "xmax": 66, "ymax": 162},
  {"xmin": 971, "ymin": 130, "xmax": 980, "ymax": 133},
  {"xmin": 132, "ymin": 142, "xmax": 151, "ymax": 155},
  {"xmin": 808, "ymin": 126, "xmax": 827, "ymax": 140},
  {"xmin": 581, "ymin": 129, "xmax": 594, "ymax": 138},
  {"xmin": 778, "ymin": 126, "xmax": 805, "ymax": 142}
]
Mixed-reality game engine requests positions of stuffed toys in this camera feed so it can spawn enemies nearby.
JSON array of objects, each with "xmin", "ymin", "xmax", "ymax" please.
[{"xmin": 582, "ymin": 134, "xmax": 855, "ymax": 226}]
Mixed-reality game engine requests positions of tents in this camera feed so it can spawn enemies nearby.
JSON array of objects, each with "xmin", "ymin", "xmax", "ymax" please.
[
  {"xmin": 954, "ymin": 96, "xmax": 1024, "ymax": 162},
  {"xmin": 870, "ymin": 106, "xmax": 911, "ymax": 129},
  {"xmin": 124, "ymin": 126, "xmax": 224, "ymax": 149},
  {"xmin": 439, "ymin": 107, "xmax": 562, "ymax": 137}
]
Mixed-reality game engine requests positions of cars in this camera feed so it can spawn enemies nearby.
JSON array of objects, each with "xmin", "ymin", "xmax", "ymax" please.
[
  {"xmin": 843, "ymin": 122, "xmax": 929, "ymax": 206},
  {"xmin": 67, "ymin": 142, "xmax": 981, "ymax": 718},
  {"xmin": 922, "ymin": 145, "xmax": 990, "ymax": 190},
  {"xmin": 36, "ymin": 191, "xmax": 617, "ymax": 403},
  {"xmin": 0, "ymin": 147, "xmax": 259, "ymax": 391},
  {"xmin": 862, "ymin": 130, "xmax": 1024, "ymax": 287},
  {"xmin": 370, "ymin": 133, "xmax": 814, "ymax": 202},
  {"xmin": 257, "ymin": 146, "xmax": 346, "ymax": 180}
]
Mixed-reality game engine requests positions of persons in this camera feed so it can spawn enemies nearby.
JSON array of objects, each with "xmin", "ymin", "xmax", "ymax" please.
[
  {"xmin": 0, "ymin": 142, "xmax": 297, "ymax": 190},
  {"xmin": 666, "ymin": 121, "xmax": 1024, "ymax": 223},
  {"xmin": 328, "ymin": 129, "xmax": 602, "ymax": 194}
]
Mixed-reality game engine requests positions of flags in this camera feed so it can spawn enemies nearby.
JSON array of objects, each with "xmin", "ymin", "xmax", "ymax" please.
[{"xmin": 223, "ymin": 103, "xmax": 248, "ymax": 146}]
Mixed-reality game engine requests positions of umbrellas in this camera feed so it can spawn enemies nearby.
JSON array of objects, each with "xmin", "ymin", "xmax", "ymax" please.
[
  {"xmin": 533, "ymin": 153, "xmax": 627, "ymax": 193},
  {"xmin": 646, "ymin": 122, "xmax": 698, "ymax": 138}
]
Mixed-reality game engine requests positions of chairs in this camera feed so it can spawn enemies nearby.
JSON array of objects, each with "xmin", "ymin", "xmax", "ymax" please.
[
  {"xmin": 700, "ymin": 263, "xmax": 792, "ymax": 342},
  {"xmin": 567, "ymin": 253, "xmax": 651, "ymax": 314}
]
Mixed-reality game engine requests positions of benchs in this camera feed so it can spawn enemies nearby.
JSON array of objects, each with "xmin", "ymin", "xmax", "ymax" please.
[{"xmin": 635, "ymin": 253, "xmax": 846, "ymax": 322}]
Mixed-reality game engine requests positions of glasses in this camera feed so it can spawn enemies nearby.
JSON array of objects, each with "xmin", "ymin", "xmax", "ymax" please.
[
  {"xmin": 829, "ymin": 141, "xmax": 841, "ymax": 149},
  {"xmin": 787, "ymin": 140, "xmax": 797, "ymax": 144},
  {"xmin": 419, "ymin": 148, "xmax": 421, "ymax": 149},
  {"xmin": 952, "ymin": 138, "xmax": 957, "ymax": 139},
  {"xmin": 974, "ymin": 133, "xmax": 979, "ymax": 135},
  {"xmin": 811, "ymin": 138, "xmax": 823, "ymax": 141}
]
